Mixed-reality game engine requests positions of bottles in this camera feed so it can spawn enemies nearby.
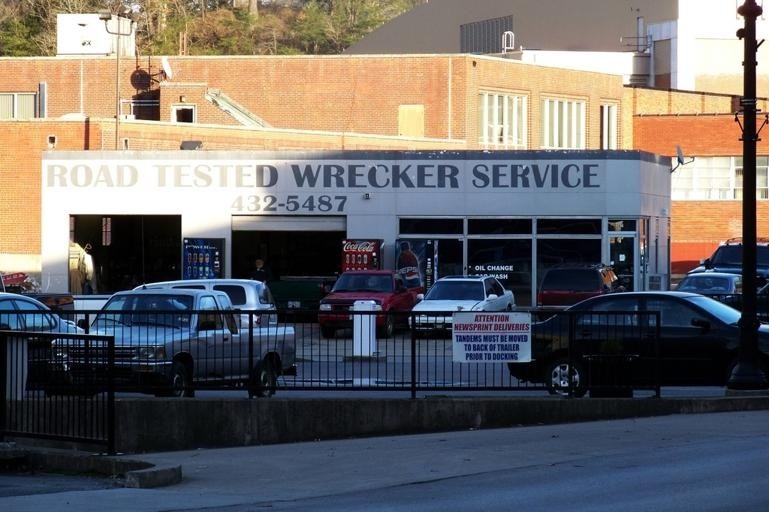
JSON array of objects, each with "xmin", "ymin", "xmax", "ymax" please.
[{"xmin": 398, "ymin": 243, "xmax": 421, "ymax": 290}]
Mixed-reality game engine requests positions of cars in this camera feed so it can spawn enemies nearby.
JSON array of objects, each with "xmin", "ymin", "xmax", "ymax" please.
[
  {"xmin": 1, "ymin": 293, "xmax": 85, "ymax": 363},
  {"xmin": 676, "ymin": 271, "xmax": 743, "ymax": 307},
  {"xmin": 408, "ymin": 275, "xmax": 515, "ymax": 336},
  {"xmin": 704, "ymin": 237, "xmax": 769, "ymax": 298},
  {"xmin": 507, "ymin": 291, "xmax": 769, "ymax": 389},
  {"xmin": 538, "ymin": 262, "xmax": 630, "ymax": 326}
]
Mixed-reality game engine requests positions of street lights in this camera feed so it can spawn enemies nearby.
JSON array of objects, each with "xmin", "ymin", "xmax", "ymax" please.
[{"xmin": 99, "ymin": 0, "xmax": 144, "ymax": 150}]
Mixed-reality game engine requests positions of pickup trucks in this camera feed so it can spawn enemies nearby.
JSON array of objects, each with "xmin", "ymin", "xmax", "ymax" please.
[
  {"xmin": 51, "ymin": 290, "xmax": 295, "ymax": 398},
  {"xmin": 319, "ymin": 270, "xmax": 427, "ymax": 339}
]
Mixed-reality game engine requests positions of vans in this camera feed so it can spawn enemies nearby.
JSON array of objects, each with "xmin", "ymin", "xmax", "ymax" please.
[{"xmin": 119, "ymin": 280, "xmax": 278, "ymax": 329}]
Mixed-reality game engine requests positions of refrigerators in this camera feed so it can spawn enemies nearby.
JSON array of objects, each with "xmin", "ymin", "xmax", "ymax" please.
[{"xmin": 395, "ymin": 240, "xmax": 439, "ymax": 298}]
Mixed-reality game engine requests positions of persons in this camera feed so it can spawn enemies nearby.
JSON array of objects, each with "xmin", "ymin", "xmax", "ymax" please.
[{"xmin": 249, "ymin": 257, "xmax": 272, "ymax": 286}]
